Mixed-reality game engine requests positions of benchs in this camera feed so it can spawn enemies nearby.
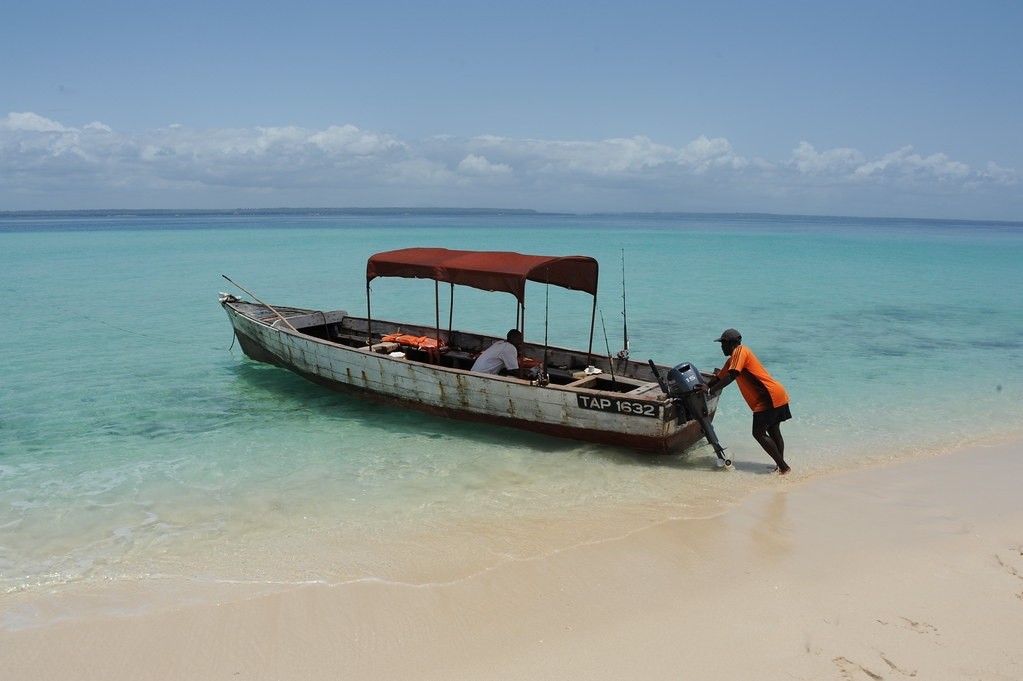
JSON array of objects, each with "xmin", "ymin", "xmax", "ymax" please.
[{"xmin": 360, "ymin": 332, "xmax": 593, "ymax": 389}]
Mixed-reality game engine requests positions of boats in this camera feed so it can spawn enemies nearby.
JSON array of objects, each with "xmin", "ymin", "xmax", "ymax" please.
[{"xmin": 217, "ymin": 248, "xmax": 724, "ymax": 456}]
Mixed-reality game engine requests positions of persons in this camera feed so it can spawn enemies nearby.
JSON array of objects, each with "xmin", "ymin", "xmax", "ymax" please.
[
  {"xmin": 469, "ymin": 329, "xmax": 524, "ymax": 379},
  {"xmin": 693, "ymin": 327, "xmax": 793, "ymax": 477}
]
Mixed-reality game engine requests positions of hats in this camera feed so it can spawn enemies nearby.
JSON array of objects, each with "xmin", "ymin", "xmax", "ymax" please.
[{"xmin": 713, "ymin": 329, "xmax": 742, "ymax": 343}]
